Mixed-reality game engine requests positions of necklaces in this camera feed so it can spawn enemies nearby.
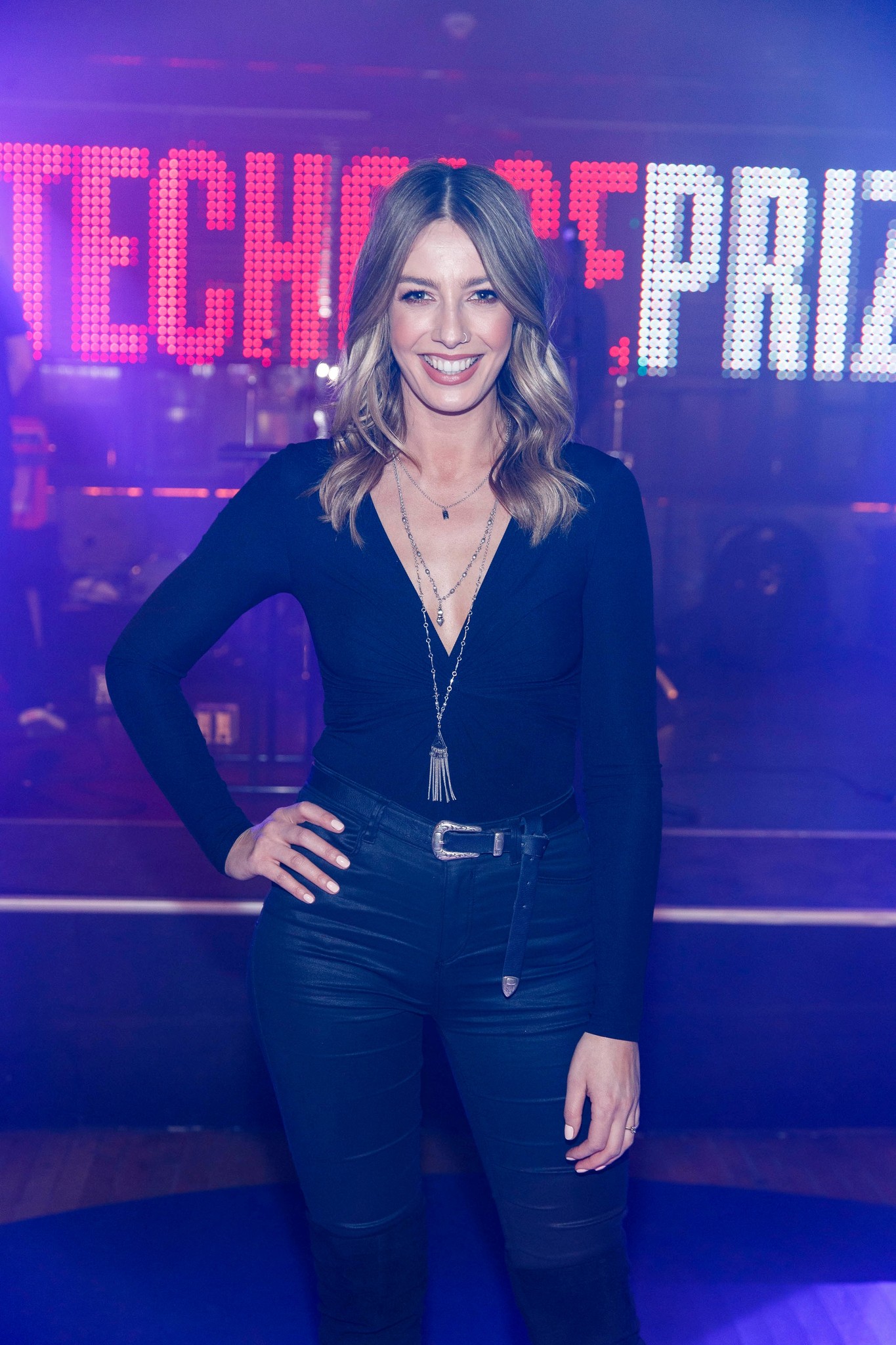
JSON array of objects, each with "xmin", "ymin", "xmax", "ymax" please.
[{"xmin": 388, "ymin": 411, "xmax": 511, "ymax": 803}]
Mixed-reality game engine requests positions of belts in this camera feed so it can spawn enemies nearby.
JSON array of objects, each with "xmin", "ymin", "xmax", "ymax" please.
[{"xmin": 306, "ymin": 762, "xmax": 578, "ymax": 998}]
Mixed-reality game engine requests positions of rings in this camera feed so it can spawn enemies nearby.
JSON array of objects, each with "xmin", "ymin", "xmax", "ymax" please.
[{"xmin": 626, "ymin": 1126, "xmax": 636, "ymax": 1133}]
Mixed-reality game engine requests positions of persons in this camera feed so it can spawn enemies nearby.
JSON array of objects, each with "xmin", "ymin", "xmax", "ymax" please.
[
  {"xmin": 0, "ymin": 257, "xmax": 72, "ymax": 744},
  {"xmin": 104, "ymin": 158, "xmax": 664, "ymax": 1344}
]
